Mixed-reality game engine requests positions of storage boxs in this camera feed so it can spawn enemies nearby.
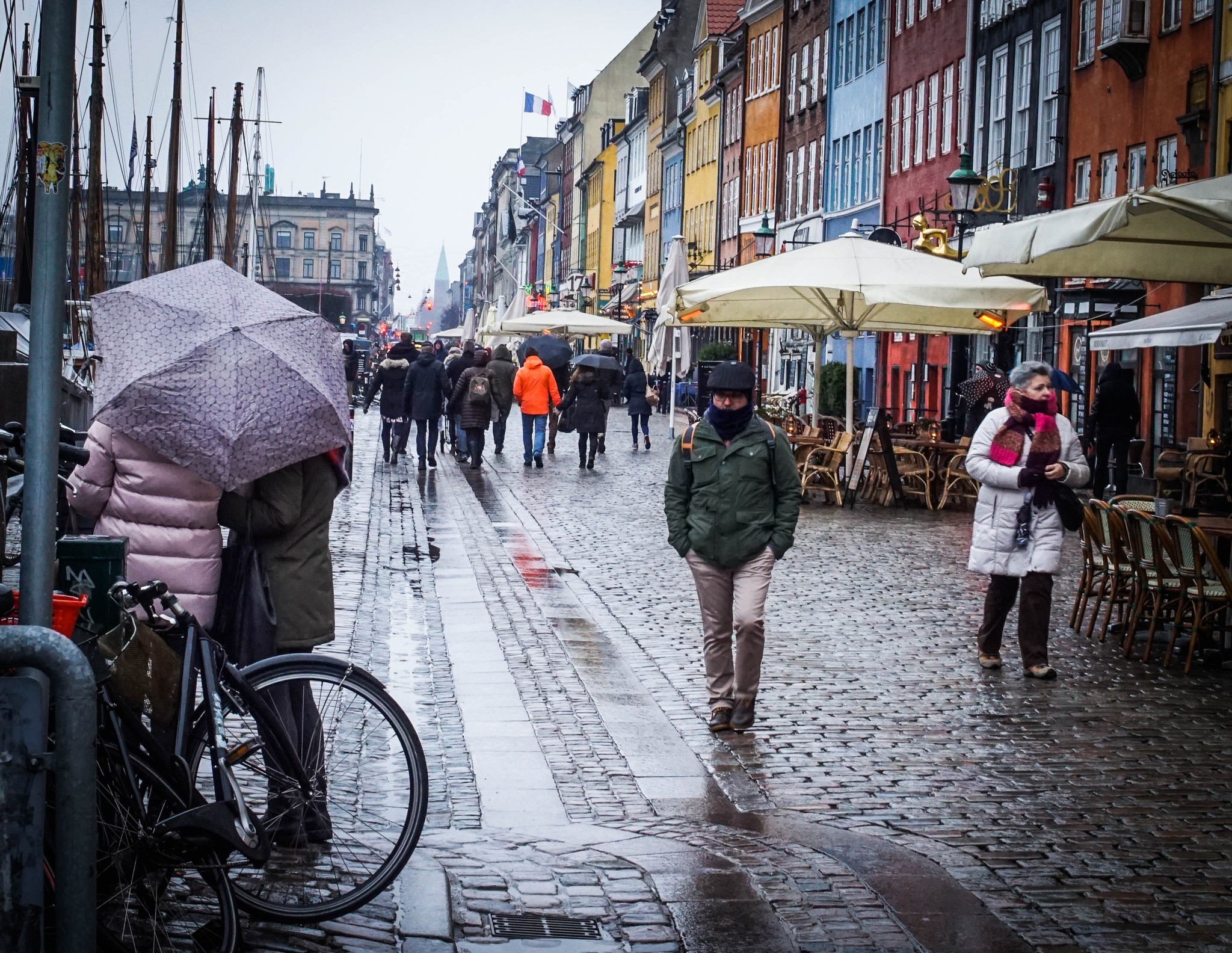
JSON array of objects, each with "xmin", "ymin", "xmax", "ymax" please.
[{"xmin": 0, "ymin": 591, "xmax": 88, "ymax": 640}]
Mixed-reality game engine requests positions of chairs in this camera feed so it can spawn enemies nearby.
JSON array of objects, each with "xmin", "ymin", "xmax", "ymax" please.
[{"xmin": 786, "ymin": 414, "xmax": 1232, "ymax": 673}]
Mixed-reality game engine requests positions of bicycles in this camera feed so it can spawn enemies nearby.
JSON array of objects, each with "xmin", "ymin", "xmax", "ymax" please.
[
  {"xmin": 0, "ymin": 419, "xmax": 89, "ymax": 568},
  {"xmin": 82, "ymin": 575, "xmax": 431, "ymax": 953}
]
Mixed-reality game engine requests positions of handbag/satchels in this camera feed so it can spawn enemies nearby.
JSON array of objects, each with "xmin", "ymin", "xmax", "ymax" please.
[
  {"xmin": 645, "ymin": 372, "xmax": 659, "ymax": 407},
  {"xmin": 211, "ymin": 498, "xmax": 276, "ymax": 698},
  {"xmin": 1047, "ymin": 479, "xmax": 1084, "ymax": 532},
  {"xmin": 558, "ymin": 390, "xmax": 576, "ymax": 433}
]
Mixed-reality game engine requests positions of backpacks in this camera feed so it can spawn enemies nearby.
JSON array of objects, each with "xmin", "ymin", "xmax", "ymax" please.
[{"xmin": 468, "ymin": 368, "xmax": 489, "ymax": 405}]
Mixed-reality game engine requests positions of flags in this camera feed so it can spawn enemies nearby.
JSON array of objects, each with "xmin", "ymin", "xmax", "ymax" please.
[{"xmin": 514, "ymin": 82, "xmax": 579, "ymax": 178}]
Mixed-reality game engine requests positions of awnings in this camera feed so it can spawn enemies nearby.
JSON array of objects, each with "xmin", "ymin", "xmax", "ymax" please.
[{"xmin": 1086, "ymin": 289, "xmax": 1232, "ymax": 350}]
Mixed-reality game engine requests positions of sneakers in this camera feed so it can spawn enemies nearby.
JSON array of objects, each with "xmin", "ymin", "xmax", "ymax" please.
[
  {"xmin": 977, "ymin": 644, "xmax": 1001, "ymax": 669},
  {"xmin": 1023, "ymin": 663, "xmax": 1057, "ymax": 679}
]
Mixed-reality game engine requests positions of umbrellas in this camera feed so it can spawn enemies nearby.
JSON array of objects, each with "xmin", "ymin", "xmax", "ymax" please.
[
  {"xmin": 569, "ymin": 354, "xmax": 624, "ymax": 370},
  {"xmin": 517, "ymin": 335, "xmax": 574, "ymax": 367},
  {"xmin": 90, "ymin": 259, "xmax": 350, "ymax": 492},
  {"xmin": 430, "ymin": 285, "xmax": 633, "ymax": 362},
  {"xmin": 669, "ymin": 232, "xmax": 1048, "ymax": 489},
  {"xmin": 1007, "ymin": 362, "xmax": 1084, "ymax": 395},
  {"xmin": 960, "ymin": 174, "xmax": 1232, "ymax": 287}
]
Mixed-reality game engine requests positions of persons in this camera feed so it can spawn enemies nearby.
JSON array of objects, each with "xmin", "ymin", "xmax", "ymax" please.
[
  {"xmin": 1091, "ymin": 349, "xmax": 1141, "ymax": 502},
  {"xmin": 663, "ymin": 361, "xmax": 803, "ymax": 732},
  {"xmin": 963, "ymin": 361, "xmax": 1092, "ymax": 675},
  {"xmin": 965, "ymin": 359, "xmax": 1005, "ymax": 437},
  {"xmin": 66, "ymin": 420, "xmax": 339, "ymax": 844},
  {"xmin": 339, "ymin": 333, "xmax": 651, "ymax": 469}
]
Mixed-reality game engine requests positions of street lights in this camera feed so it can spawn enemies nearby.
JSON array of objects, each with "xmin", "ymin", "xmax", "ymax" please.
[
  {"xmin": 946, "ymin": 151, "xmax": 985, "ymax": 464},
  {"xmin": 752, "ymin": 208, "xmax": 779, "ymax": 413},
  {"xmin": 397, "ymin": 333, "xmax": 399, "ymax": 341},
  {"xmin": 382, "ymin": 328, "xmax": 386, "ymax": 342},
  {"xmin": 613, "ymin": 255, "xmax": 628, "ymax": 365},
  {"xmin": 339, "ymin": 311, "xmax": 347, "ymax": 333}
]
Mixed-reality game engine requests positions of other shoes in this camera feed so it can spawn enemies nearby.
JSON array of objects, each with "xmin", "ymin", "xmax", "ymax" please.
[
  {"xmin": 257, "ymin": 811, "xmax": 306, "ymax": 847},
  {"xmin": 525, "ymin": 433, "xmax": 651, "ymax": 469},
  {"xmin": 302, "ymin": 799, "xmax": 332, "ymax": 840},
  {"xmin": 382, "ymin": 435, "xmax": 504, "ymax": 469}
]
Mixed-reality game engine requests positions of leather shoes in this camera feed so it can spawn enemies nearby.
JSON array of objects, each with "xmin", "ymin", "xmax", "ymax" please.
[
  {"xmin": 730, "ymin": 701, "xmax": 755, "ymax": 728},
  {"xmin": 709, "ymin": 707, "xmax": 732, "ymax": 731}
]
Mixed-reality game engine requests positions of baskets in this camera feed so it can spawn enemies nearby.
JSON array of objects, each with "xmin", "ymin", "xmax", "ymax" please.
[{"xmin": 0, "ymin": 591, "xmax": 197, "ymax": 722}]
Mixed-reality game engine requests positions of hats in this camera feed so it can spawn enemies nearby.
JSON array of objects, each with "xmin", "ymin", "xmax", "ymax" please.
[
  {"xmin": 975, "ymin": 359, "xmax": 997, "ymax": 373},
  {"xmin": 421, "ymin": 341, "xmax": 433, "ymax": 348},
  {"xmin": 599, "ymin": 339, "xmax": 613, "ymax": 349},
  {"xmin": 707, "ymin": 360, "xmax": 756, "ymax": 390},
  {"xmin": 1104, "ymin": 363, "xmax": 1121, "ymax": 373}
]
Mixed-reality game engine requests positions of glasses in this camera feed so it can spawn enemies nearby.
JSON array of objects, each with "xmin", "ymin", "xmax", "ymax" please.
[{"xmin": 714, "ymin": 391, "xmax": 745, "ymax": 402}]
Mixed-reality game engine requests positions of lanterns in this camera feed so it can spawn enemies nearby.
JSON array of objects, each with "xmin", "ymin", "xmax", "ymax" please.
[{"xmin": 381, "ymin": 302, "xmax": 433, "ymax": 349}]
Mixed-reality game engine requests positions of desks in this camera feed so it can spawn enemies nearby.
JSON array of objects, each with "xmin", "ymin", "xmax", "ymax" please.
[
  {"xmin": 1185, "ymin": 517, "xmax": 1232, "ymax": 535},
  {"xmin": 787, "ymin": 436, "xmax": 824, "ymax": 454}
]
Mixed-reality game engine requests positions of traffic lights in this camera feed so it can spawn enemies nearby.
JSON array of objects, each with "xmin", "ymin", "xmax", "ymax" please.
[{"xmin": 428, "ymin": 303, "xmax": 432, "ymax": 311}]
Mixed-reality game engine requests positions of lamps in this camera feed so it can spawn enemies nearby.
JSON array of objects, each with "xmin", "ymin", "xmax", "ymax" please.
[
  {"xmin": 887, "ymin": 414, "xmax": 893, "ymax": 434},
  {"xmin": 786, "ymin": 415, "xmax": 797, "ymax": 437},
  {"xmin": 928, "ymin": 420, "xmax": 941, "ymax": 443},
  {"xmin": 1206, "ymin": 428, "xmax": 1220, "ymax": 452}
]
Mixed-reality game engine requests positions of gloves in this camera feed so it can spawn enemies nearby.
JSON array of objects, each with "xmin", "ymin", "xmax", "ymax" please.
[
  {"xmin": 364, "ymin": 406, "xmax": 370, "ymax": 414},
  {"xmin": 1083, "ymin": 431, "xmax": 1095, "ymax": 448},
  {"xmin": 1033, "ymin": 477, "xmax": 1053, "ymax": 509},
  {"xmin": 552, "ymin": 407, "xmax": 562, "ymax": 415},
  {"xmin": 1129, "ymin": 427, "xmax": 1135, "ymax": 441},
  {"xmin": 1020, "ymin": 468, "xmax": 1046, "ymax": 486}
]
